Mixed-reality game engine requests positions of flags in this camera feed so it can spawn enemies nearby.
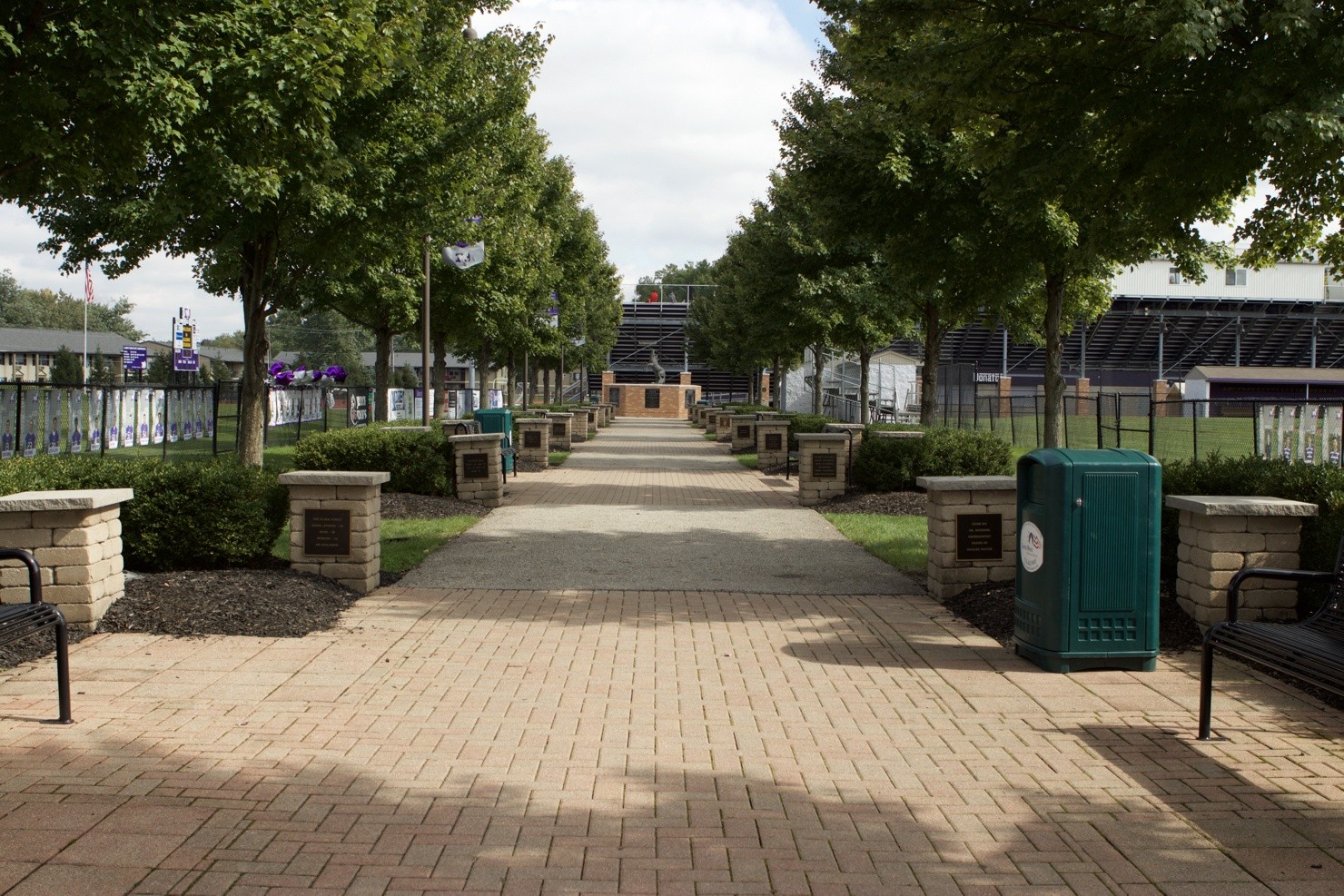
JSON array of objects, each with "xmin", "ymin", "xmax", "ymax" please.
[
  {"xmin": 442, "ymin": 218, "xmax": 484, "ymax": 269},
  {"xmin": 85, "ymin": 263, "xmax": 94, "ymax": 303}
]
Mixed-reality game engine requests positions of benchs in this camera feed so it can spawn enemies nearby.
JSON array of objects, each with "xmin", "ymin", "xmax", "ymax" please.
[
  {"xmin": 787, "ymin": 426, "xmax": 852, "ymax": 486},
  {"xmin": 1195, "ymin": 541, "xmax": 1344, "ymax": 741},
  {"xmin": 0, "ymin": 548, "xmax": 74, "ymax": 725},
  {"xmin": 455, "ymin": 420, "xmax": 519, "ymax": 484}
]
